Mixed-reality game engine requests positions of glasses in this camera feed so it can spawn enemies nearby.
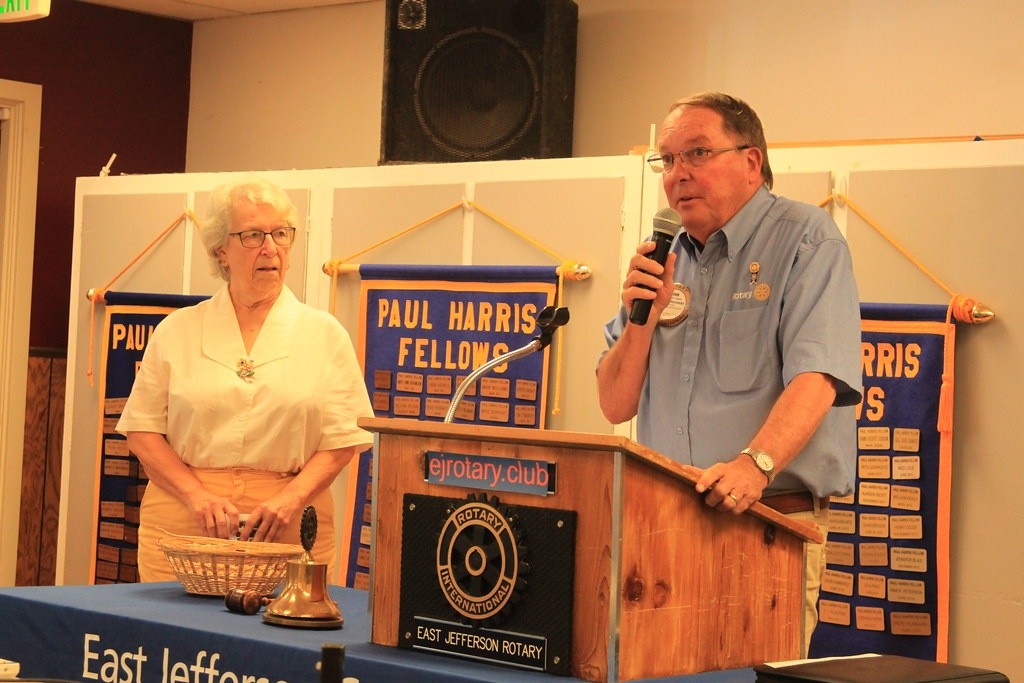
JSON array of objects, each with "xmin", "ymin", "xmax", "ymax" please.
[
  {"xmin": 647, "ymin": 145, "xmax": 752, "ymax": 174},
  {"xmin": 229, "ymin": 226, "xmax": 296, "ymax": 248}
]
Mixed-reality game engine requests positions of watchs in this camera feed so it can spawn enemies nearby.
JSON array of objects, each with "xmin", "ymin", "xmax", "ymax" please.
[{"xmin": 740, "ymin": 448, "xmax": 774, "ymax": 485}]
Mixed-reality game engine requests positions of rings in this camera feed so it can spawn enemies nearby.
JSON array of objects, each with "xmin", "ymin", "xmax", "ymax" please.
[{"xmin": 729, "ymin": 494, "xmax": 738, "ymax": 503}]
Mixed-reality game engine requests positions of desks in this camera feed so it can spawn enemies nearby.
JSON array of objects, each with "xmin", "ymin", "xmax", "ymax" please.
[{"xmin": 0, "ymin": 580, "xmax": 762, "ymax": 683}]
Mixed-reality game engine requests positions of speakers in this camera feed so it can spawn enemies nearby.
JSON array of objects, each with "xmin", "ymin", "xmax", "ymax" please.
[{"xmin": 381, "ymin": 0, "xmax": 578, "ymax": 164}]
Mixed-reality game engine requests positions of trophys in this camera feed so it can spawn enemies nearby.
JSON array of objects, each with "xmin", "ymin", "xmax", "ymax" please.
[{"xmin": 262, "ymin": 505, "xmax": 342, "ymax": 627}]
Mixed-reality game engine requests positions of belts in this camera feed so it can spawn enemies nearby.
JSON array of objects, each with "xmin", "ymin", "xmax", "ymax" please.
[{"xmin": 757, "ymin": 488, "xmax": 831, "ymax": 515}]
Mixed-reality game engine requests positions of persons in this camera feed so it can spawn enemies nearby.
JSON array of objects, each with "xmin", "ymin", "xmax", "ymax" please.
[
  {"xmin": 596, "ymin": 92, "xmax": 862, "ymax": 663},
  {"xmin": 115, "ymin": 179, "xmax": 376, "ymax": 584}
]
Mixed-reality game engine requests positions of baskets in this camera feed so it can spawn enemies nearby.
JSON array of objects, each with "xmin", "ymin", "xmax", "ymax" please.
[{"xmin": 157, "ymin": 535, "xmax": 305, "ymax": 597}]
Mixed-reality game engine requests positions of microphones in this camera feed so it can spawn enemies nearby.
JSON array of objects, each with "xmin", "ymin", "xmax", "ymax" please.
[{"xmin": 629, "ymin": 207, "xmax": 683, "ymax": 326}]
